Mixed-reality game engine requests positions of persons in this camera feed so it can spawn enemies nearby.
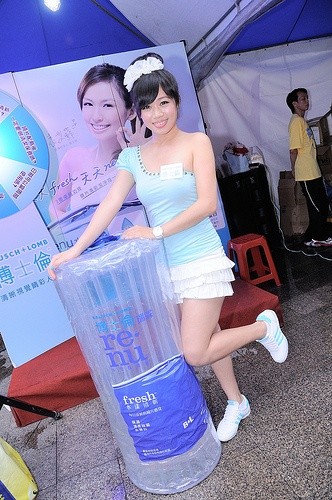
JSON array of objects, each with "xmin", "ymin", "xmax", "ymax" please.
[
  {"xmin": 45, "ymin": 53, "xmax": 289, "ymax": 442},
  {"xmin": 285, "ymin": 87, "xmax": 332, "ymax": 247},
  {"xmin": 47, "ymin": 62, "xmax": 154, "ymax": 220}
]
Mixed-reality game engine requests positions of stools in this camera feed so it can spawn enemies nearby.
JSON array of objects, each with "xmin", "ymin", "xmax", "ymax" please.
[{"xmin": 228, "ymin": 233, "xmax": 282, "ymax": 287}]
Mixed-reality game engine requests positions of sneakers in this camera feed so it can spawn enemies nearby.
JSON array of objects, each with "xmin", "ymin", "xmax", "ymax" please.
[
  {"xmin": 304, "ymin": 241, "xmax": 312, "ymax": 246},
  {"xmin": 311, "ymin": 237, "xmax": 332, "ymax": 247},
  {"xmin": 256, "ymin": 309, "xmax": 288, "ymax": 363},
  {"xmin": 217, "ymin": 394, "xmax": 250, "ymax": 441}
]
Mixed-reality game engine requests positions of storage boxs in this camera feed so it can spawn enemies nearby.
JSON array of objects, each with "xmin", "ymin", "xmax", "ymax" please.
[{"xmin": 278, "ymin": 116, "xmax": 332, "ymax": 235}]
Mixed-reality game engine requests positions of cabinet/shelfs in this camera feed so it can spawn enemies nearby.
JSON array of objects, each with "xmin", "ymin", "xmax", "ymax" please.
[{"xmin": 217, "ymin": 163, "xmax": 280, "ymax": 256}]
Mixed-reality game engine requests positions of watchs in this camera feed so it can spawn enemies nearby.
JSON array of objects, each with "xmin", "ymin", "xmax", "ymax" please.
[{"xmin": 152, "ymin": 226, "xmax": 163, "ymax": 239}]
